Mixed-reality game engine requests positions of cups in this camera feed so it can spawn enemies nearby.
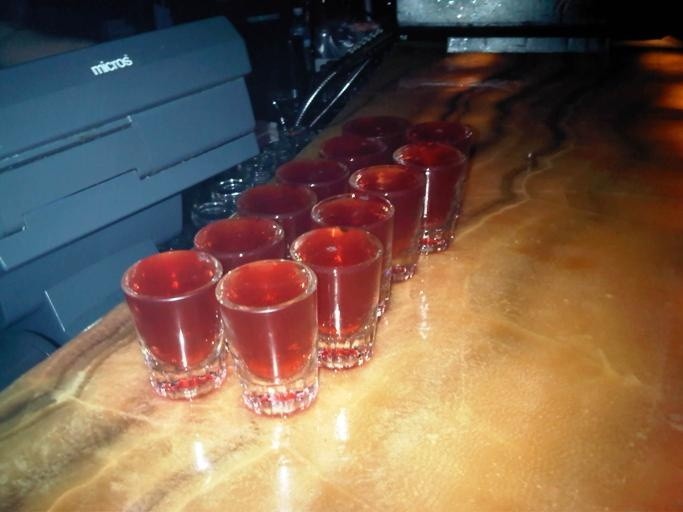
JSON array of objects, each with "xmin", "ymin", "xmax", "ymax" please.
[
  {"xmin": 312, "ymin": 194, "xmax": 392, "ymax": 318},
  {"xmin": 277, "ymin": 161, "xmax": 348, "ymax": 201},
  {"xmin": 214, "ymin": 259, "xmax": 319, "ymax": 416},
  {"xmin": 288, "ymin": 227, "xmax": 383, "ymax": 370},
  {"xmin": 393, "ymin": 144, "xmax": 469, "ymax": 253},
  {"xmin": 319, "ymin": 137, "xmax": 389, "ymax": 177},
  {"xmin": 238, "ymin": 184, "xmax": 316, "ymax": 252},
  {"xmin": 408, "ymin": 121, "xmax": 475, "ymax": 168},
  {"xmin": 119, "ymin": 250, "xmax": 231, "ymax": 400},
  {"xmin": 342, "ymin": 115, "xmax": 411, "ymax": 164},
  {"xmin": 348, "ymin": 166, "xmax": 428, "ymax": 280},
  {"xmin": 195, "ymin": 218, "xmax": 286, "ymax": 275}
]
